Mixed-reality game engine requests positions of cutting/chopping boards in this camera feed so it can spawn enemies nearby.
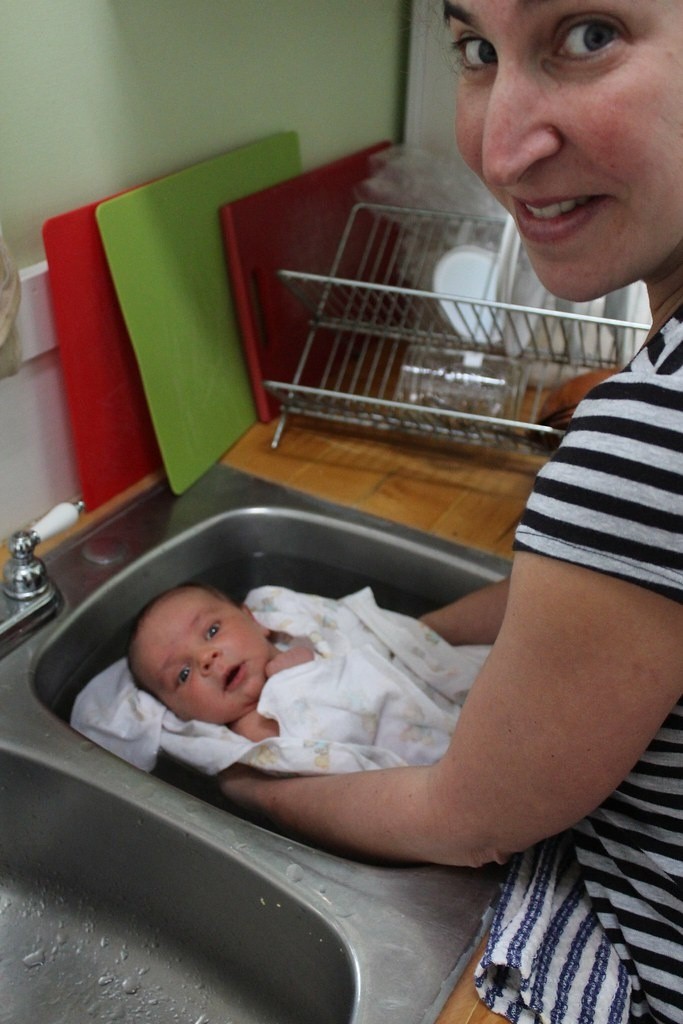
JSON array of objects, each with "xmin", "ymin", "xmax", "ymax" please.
[
  {"xmin": 220, "ymin": 142, "xmax": 404, "ymax": 425},
  {"xmin": 43, "ymin": 180, "xmax": 162, "ymax": 512},
  {"xmin": 94, "ymin": 128, "xmax": 306, "ymax": 494}
]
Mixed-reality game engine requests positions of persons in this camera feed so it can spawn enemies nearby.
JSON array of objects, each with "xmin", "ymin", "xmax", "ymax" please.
[
  {"xmin": 214, "ymin": 0, "xmax": 683, "ymax": 1024},
  {"xmin": 128, "ymin": 581, "xmax": 495, "ymax": 773}
]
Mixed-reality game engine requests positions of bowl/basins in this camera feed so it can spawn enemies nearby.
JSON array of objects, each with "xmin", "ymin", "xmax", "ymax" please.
[{"xmin": 528, "ymin": 368, "xmax": 620, "ymax": 448}]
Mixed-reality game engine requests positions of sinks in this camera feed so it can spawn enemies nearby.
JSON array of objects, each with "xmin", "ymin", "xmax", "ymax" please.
[
  {"xmin": 0, "ymin": 736, "xmax": 385, "ymax": 1024},
  {"xmin": 26, "ymin": 492, "xmax": 541, "ymax": 906}
]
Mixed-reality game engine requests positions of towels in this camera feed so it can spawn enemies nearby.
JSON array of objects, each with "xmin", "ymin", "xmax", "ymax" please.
[{"xmin": 474, "ymin": 835, "xmax": 632, "ymax": 1024}]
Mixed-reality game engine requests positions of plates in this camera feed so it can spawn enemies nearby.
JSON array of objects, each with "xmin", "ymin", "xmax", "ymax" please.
[{"xmin": 431, "ymin": 213, "xmax": 605, "ymax": 358}]
